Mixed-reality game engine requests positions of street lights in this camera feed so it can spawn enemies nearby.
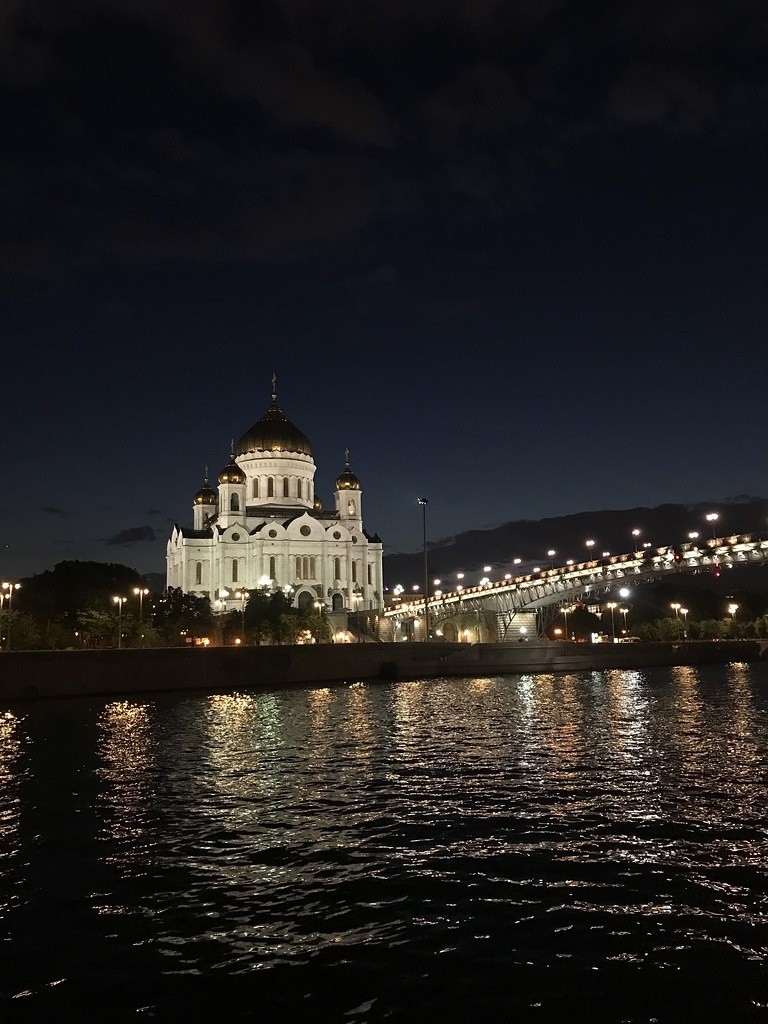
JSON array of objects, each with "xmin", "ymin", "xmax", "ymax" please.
[
  {"xmin": 457, "ymin": 574, "xmax": 464, "ymax": 586},
  {"xmin": 586, "ymin": 540, "xmax": 595, "ymax": 561},
  {"xmin": 314, "ymin": 599, "xmax": 326, "ymax": 643},
  {"xmin": 680, "ymin": 609, "xmax": 689, "ymax": 642},
  {"xmin": 417, "ymin": 497, "xmax": 430, "ymax": 641},
  {"xmin": 514, "ymin": 559, "xmax": 521, "ymax": 577},
  {"xmin": 632, "ymin": 530, "xmax": 640, "ymax": 552},
  {"xmin": 394, "ymin": 589, "xmax": 399, "ymax": 605},
  {"xmin": 113, "ymin": 597, "xmax": 127, "ymax": 648},
  {"xmin": 620, "ymin": 609, "xmax": 629, "ymax": 637},
  {"xmin": 134, "ymin": 588, "xmax": 149, "ymax": 647},
  {"xmin": 236, "ymin": 587, "xmax": 249, "ymax": 645},
  {"xmin": 607, "ymin": 603, "xmax": 617, "ymax": 642},
  {"xmin": 728, "ymin": 604, "xmax": 738, "ymax": 640},
  {"xmin": 0, "ymin": 582, "xmax": 21, "ymax": 650},
  {"xmin": 484, "ymin": 566, "xmax": 492, "ymax": 578},
  {"xmin": 434, "ymin": 579, "xmax": 441, "ymax": 590},
  {"xmin": 548, "ymin": 550, "xmax": 555, "ymax": 569},
  {"xmin": 352, "ymin": 595, "xmax": 363, "ymax": 641},
  {"xmin": 413, "ymin": 585, "xmax": 419, "ymax": 600},
  {"xmin": 560, "ymin": 608, "xmax": 569, "ymax": 640},
  {"xmin": 706, "ymin": 514, "xmax": 719, "ymax": 539},
  {"xmin": 671, "ymin": 604, "xmax": 681, "ymax": 641}
]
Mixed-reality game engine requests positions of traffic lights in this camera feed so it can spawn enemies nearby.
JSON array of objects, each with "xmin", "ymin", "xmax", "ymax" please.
[
  {"xmin": 714, "ymin": 566, "xmax": 722, "ymax": 578},
  {"xmin": 674, "ymin": 554, "xmax": 682, "ymax": 563}
]
